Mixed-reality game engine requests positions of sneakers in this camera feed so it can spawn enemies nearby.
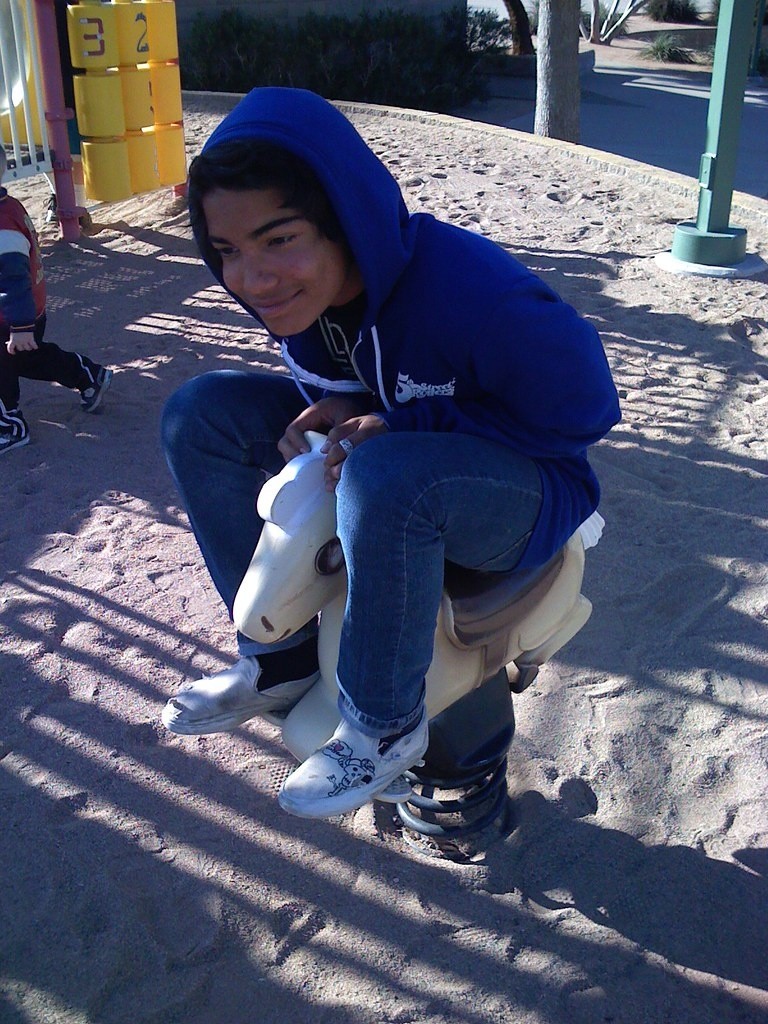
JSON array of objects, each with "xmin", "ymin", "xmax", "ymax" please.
[
  {"xmin": 0, "ymin": 408, "xmax": 30, "ymax": 455},
  {"xmin": 279, "ymin": 708, "xmax": 429, "ymax": 819},
  {"xmin": 81, "ymin": 367, "xmax": 113, "ymax": 412},
  {"xmin": 162, "ymin": 655, "xmax": 320, "ymax": 735}
]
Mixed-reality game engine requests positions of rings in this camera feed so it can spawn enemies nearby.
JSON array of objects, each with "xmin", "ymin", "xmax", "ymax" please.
[{"xmin": 339, "ymin": 438, "xmax": 353, "ymax": 456}]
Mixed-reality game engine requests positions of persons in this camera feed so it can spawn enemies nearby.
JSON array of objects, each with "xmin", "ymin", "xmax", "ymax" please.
[
  {"xmin": 0, "ymin": 146, "xmax": 113, "ymax": 454},
  {"xmin": 160, "ymin": 87, "xmax": 621, "ymax": 821}
]
